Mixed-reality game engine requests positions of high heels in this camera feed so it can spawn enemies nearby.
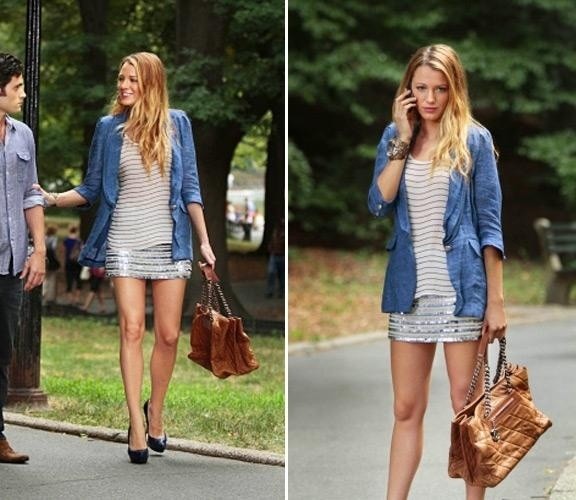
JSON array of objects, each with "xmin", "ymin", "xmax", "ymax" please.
[
  {"xmin": 143, "ymin": 400, "xmax": 167, "ymax": 452},
  {"xmin": 127, "ymin": 425, "xmax": 148, "ymax": 464}
]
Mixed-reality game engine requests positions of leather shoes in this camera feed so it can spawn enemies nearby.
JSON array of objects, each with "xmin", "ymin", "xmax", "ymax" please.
[{"xmin": 0, "ymin": 438, "xmax": 29, "ymax": 464}]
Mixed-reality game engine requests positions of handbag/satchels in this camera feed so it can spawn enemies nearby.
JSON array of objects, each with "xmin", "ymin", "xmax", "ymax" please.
[
  {"xmin": 187, "ymin": 262, "xmax": 260, "ymax": 379},
  {"xmin": 447, "ymin": 326, "xmax": 553, "ymax": 487}
]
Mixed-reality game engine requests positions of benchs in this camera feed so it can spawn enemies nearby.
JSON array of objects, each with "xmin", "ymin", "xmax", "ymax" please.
[{"xmin": 534, "ymin": 217, "xmax": 576, "ymax": 306}]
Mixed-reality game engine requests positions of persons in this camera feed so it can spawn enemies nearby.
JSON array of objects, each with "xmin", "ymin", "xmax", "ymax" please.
[
  {"xmin": 43, "ymin": 228, "xmax": 107, "ymax": 313},
  {"xmin": 264, "ymin": 223, "xmax": 285, "ymax": 299},
  {"xmin": 0, "ymin": 53, "xmax": 49, "ymax": 463},
  {"xmin": 368, "ymin": 42, "xmax": 507, "ymax": 500},
  {"xmin": 33, "ymin": 52, "xmax": 216, "ymax": 463},
  {"xmin": 229, "ymin": 198, "xmax": 258, "ymax": 241}
]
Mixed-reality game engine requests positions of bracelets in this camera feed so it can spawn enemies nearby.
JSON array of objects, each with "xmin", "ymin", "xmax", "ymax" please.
[
  {"xmin": 51, "ymin": 193, "xmax": 59, "ymax": 201},
  {"xmin": 386, "ymin": 138, "xmax": 408, "ymax": 159}
]
